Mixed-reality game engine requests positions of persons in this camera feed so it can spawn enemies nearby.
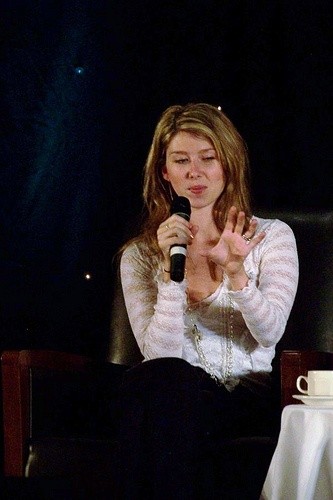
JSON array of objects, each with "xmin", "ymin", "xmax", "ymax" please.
[{"xmin": 120, "ymin": 103, "xmax": 299, "ymax": 500}]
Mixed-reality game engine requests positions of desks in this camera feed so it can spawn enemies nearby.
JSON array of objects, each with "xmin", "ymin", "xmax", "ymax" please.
[{"xmin": 259, "ymin": 405, "xmax": 333, "ymax": 500}]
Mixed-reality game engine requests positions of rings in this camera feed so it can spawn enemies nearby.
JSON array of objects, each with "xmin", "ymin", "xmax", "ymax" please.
[
  {"xmin": 242, "ymin": 234, "xmax": 251, "ymax": 242},
  {"xmin": 166, "ymin": 223, "xmax": 169, "ymax": 230}
]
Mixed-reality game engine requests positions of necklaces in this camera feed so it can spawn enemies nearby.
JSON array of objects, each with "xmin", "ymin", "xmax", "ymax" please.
[{"xmin": 183, "ymin": 270, "xmax": 235, "ymax": 384}]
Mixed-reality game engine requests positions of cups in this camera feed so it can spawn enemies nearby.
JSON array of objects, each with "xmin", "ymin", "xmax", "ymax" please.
[{"xmin": 297, "ymin": 370, "xmax": 333, "ymax": 396}]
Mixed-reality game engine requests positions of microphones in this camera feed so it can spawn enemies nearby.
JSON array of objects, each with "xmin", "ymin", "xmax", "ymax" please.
[{"xmin": 169, "ymin": 196, "xmax": 191, "ymax": 283}]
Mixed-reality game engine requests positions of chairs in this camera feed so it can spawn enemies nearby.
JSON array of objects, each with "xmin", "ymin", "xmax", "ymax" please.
[{"xmin": 1, "ymin": 213, "xmax": 333, "ymax": 476}]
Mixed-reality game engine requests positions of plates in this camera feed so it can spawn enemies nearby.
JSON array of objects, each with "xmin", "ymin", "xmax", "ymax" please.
[{"xmin": 293, "ymin": 395, "xmax": 333, "ymax": 408}]
